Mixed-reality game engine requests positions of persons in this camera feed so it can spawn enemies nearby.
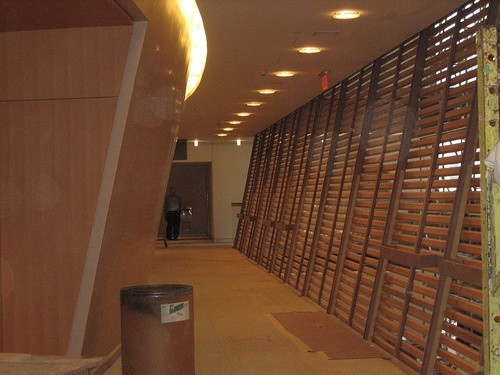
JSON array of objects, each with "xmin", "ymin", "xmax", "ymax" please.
[{"xmin": 164, "ymin": 187, "xmax": 181, "ymax": 240}]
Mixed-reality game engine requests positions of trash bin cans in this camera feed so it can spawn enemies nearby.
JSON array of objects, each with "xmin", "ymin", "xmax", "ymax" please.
[{"xmin": 119, "ymin": 283, "xmax": 196, "ymax": 375}]
